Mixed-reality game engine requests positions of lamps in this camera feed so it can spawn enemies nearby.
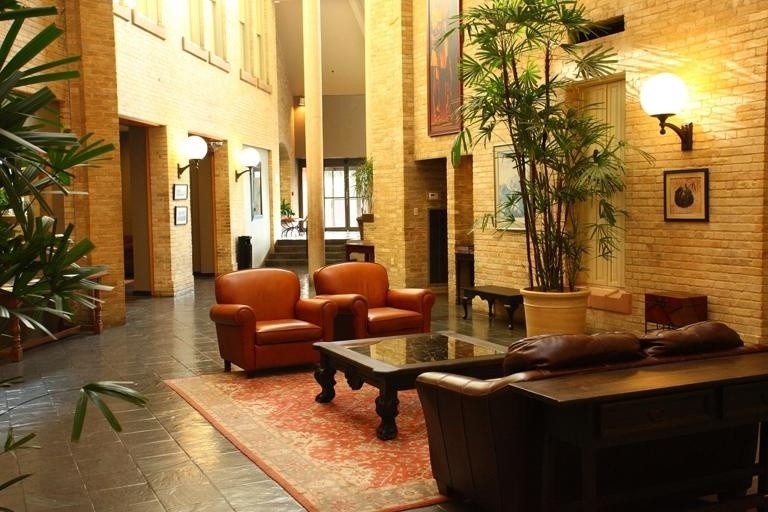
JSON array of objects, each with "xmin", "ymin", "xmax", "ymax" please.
[
  {"xmin": 178, "ymin": 135, "xmax": 208, "ymax": 176},
  {"xmin": 295, "ymin": 96, "xmax": 305, "ymax": 106},
  {"xmin": 640, "ymin": 73, "xmax": 692, "ymax": 152},
  {"xmin": 235, "ymin": 148, "xmax": 261, "ymax": 181}
]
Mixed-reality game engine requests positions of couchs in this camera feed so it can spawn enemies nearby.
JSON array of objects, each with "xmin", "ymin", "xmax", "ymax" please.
[
  {"xmin": 312, "ymin": 263, "xmax": 435, "ymax": 337},
  {"xmin": 209, "ymin": 268, "xmax": 336, "ymax": 378},
  {"xmin": 417, "ymin": 321, "xmax": 768, "ymax": 512}
]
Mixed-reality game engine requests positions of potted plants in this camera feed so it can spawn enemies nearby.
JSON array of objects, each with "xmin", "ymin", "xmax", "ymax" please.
[
  {"xmin": 351, "ymin": 156, "xmax": 374, "ymax": 240},
  {"xmin": 436, "ymin": 0, "xmax": 654, "ymax": 336}
]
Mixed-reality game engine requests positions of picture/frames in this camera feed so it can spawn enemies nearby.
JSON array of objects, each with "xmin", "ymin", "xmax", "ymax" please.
[
  {"xmin": 493, "ymin": 143, "xmax": 534, "ymax": 232},
  {"xmin": 175, "ymin": 183, "xmax": 188, "ymax": 201},
  {"xmin": 663, "ymin": 170, "xmax": 711, "ymax": 221},
  {"xmin": 175, "ymin": 205, "xmax": 188, "ymax": 225},
  {"xmin": 426, "ymin": 0, "xmax": 464, "ymax": 137}
]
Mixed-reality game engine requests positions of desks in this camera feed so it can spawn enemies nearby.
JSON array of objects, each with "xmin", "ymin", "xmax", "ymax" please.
[
  {"xmin": 510, "ymin": 350, "xmax": 767, "ymax": 512},
  {"xmin": 461, "ymin": 285, "xmax": 523, "ymax": 330}
]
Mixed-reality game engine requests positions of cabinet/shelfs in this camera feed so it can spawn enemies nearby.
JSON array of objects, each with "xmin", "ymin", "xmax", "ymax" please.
[
  {"xmin": 456, "ymin": 254, "xmax": 474, "ymax": 303},
  {"xmin": 644, "ymin": 291, "xmax": 708, "ymax": 333},
  {"xmin": 345, "ymin": 242, "xmax": 375, "ymax": 263}
]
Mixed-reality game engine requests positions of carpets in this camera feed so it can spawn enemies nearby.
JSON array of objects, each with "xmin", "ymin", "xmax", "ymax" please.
[{"xmin": 161, "ymin": 367, "xmax": 448, "ymax": 511}]
